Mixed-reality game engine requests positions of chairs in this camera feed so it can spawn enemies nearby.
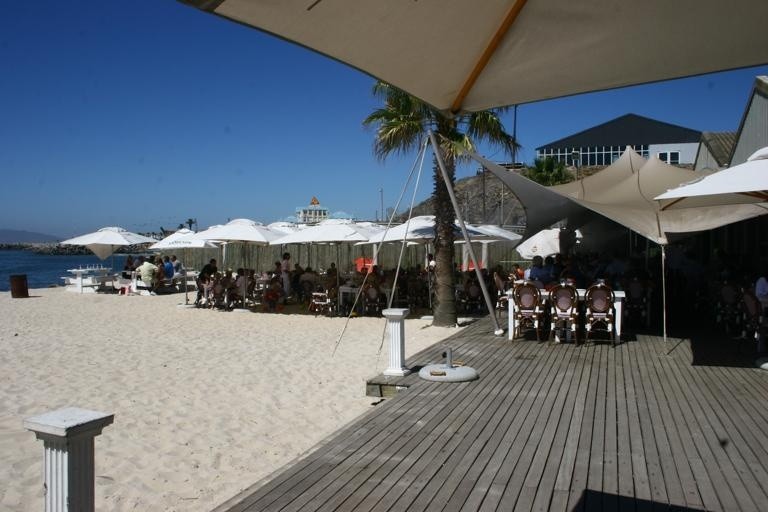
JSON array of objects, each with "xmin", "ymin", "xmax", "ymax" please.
[
  {"xmin": 667, "ymin": 268, "xmax": 768, "ymax": 364},
  {"xmin": 548, "ymin": 284, "xmax": 579, "ymax": 347},
  {"xmin": 584, "ymin": 283, "xmax": 616, "ymax": 348},
  {"xmin": 63, "ymin": 268, "xmax": 653, "ymax": 328},
  {"xmin": 512, "ymin": 281, "xmax": 543, "ymax": 343}
]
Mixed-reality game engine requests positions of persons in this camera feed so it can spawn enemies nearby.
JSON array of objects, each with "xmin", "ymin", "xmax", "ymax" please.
[
  {"xmin": 753, "ymin": 273, "xmax": 768, "ymax": 313},
  {"xmin": 528, "ymin": 245, "xmax": 647, "ymax": 315},
  {"xmin": 120, "ymin": 253, "xmax": 182, "ymax": 296}
]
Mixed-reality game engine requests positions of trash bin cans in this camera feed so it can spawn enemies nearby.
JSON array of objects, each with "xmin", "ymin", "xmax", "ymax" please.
[{"xmin": 9, "ymin": 275, "xmax": 29, "ymax": 298}]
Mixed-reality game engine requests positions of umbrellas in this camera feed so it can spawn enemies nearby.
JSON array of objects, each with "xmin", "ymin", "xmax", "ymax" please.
[
  {"xmin": 57, "ymin": 226, "xmax": 159, "ymax": 294},
  {"xmin": 651, "ymin": 147, "xmax": 768, "ymax": 203},
  {"xmin": 146, "ymin": 228, "xmax": 218, "ymax": 305}
]
